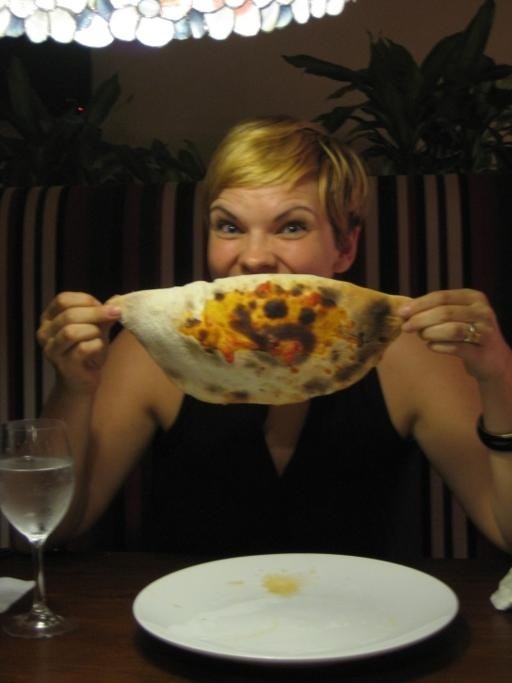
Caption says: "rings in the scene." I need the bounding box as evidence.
[461,320,480,344]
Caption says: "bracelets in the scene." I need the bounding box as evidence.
[474,412,512,455]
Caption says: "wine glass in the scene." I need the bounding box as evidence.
[0,416,76,639]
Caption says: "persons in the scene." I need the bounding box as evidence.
[6,110,512,560]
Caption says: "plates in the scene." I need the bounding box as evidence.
[132,552,460,665]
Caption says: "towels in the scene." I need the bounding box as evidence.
[0,576,36,614]
[490,568,512,611]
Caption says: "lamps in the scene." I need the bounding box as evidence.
[0,0,345,48]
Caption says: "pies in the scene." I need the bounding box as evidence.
[106,273,415,405]
